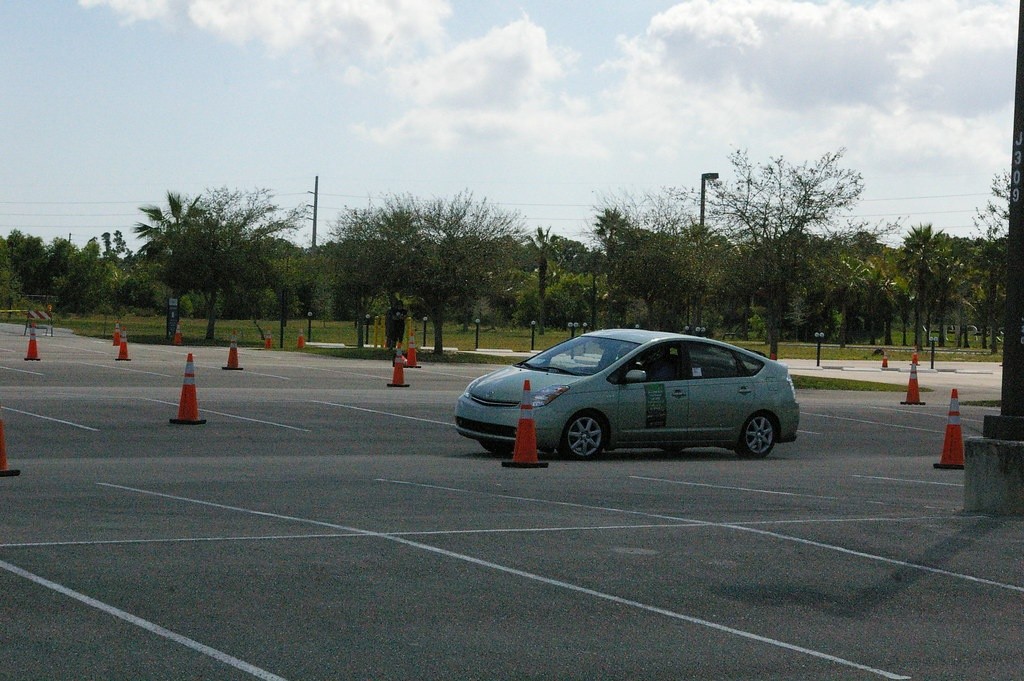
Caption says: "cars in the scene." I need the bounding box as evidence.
[453,328,803,461]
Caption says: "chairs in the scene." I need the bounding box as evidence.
[617,347,636,370]
[645,354,684,383]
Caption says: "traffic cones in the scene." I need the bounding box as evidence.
[386,343,410,388]
[404,331,422,369]
[501,379,549,468]
[221,330,243,371]
[297,328,305,348]
[0,418,20,476]
[881,347,890,370]
[933,389,965,469]
[264,327,272,350]
[170,353,207,424]
[23,323,41,361]
[112,321,120,347]
[115,327,132,361]
[172,321,185,346]
[899,353,926,405]
[392,352,421,369]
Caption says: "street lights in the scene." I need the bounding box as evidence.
[699,172,719,226]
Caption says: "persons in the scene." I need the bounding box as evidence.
[642,346,673,380]
[385,300,408,349]
[616,344,637,369]
